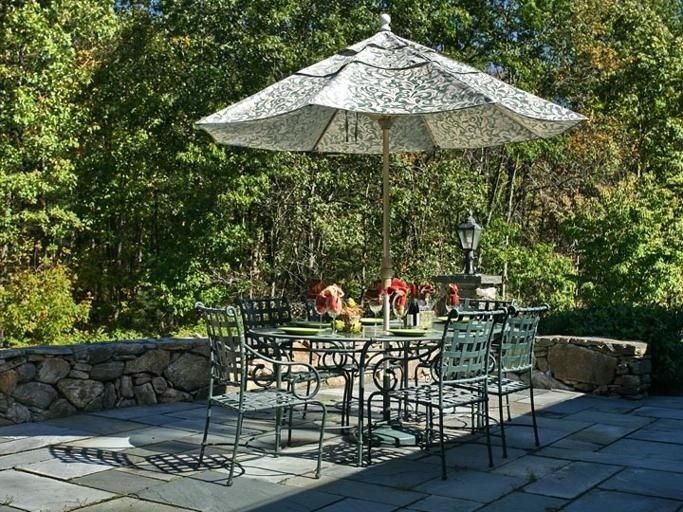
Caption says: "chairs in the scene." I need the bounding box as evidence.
[236,295,349,446]
[301,292,404,429]
[366,306,494,479]
[422,302,550,457]
[193,302,327,488]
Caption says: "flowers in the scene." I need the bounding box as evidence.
[311,278,364,333]
[366,273,460,305]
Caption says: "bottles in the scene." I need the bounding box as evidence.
[407,284,420,327]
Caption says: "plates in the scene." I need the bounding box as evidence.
[292,321,333,328]
[359,317,383,324]
[384,328,428,337]
[438,317,470,323]
[276,327,325,336]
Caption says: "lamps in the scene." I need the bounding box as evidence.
[454,208,485,275]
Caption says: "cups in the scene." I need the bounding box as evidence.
[445,295,459,313]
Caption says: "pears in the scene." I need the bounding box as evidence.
[332,320,345,331]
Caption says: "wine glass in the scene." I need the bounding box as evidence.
[367,293,383,330]
[392,293,409,329]
[327,295,343,338]
[314,294,328,329]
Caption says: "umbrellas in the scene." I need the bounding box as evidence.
[193,13,589,446]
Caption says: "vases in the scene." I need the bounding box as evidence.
[403,309,437,331]
[334,324,363,338]
